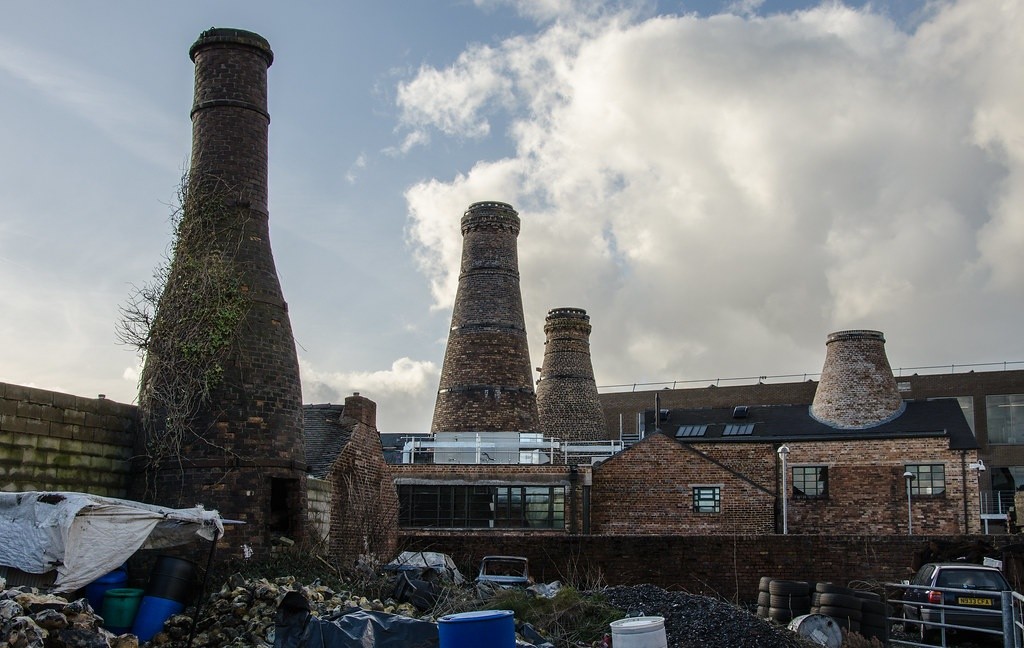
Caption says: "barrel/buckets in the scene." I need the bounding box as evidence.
[788,612,842,648]
[437,609,516,648]
[610,616,667,648]
[78,556,200,643]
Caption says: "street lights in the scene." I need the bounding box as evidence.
[903,471,915,535]
[776,444,791,534]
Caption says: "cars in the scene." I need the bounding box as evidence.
[901,562,1012,643]
[384,552,461,587]
[474,555,529,591]
